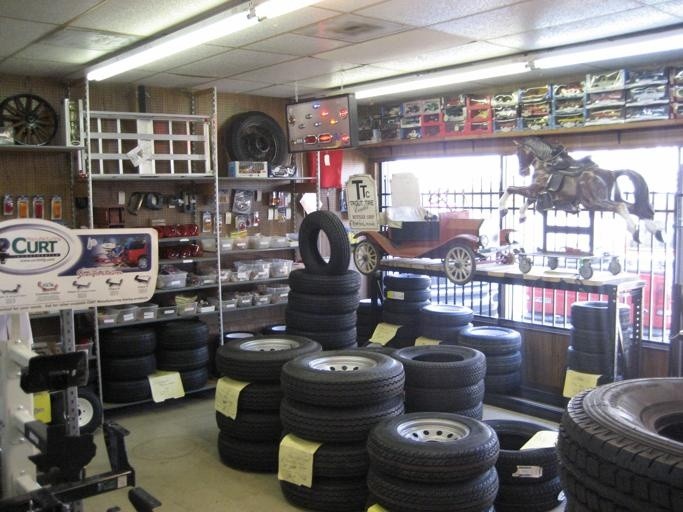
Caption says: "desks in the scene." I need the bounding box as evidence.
[374,253,646,424]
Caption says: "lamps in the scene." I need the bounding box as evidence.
[313,24,683,104]
[59,1,317,79]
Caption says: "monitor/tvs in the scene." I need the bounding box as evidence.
[285,92,360,155]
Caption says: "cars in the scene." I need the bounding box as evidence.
[114,241,150,270]
[353,169,524,286]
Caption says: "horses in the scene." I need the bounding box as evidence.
[495,135,666,247]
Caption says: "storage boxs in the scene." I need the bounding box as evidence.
[226,158,269,178]
[76,230,305,334]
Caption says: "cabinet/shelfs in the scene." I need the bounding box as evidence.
[76,80,325,411]
